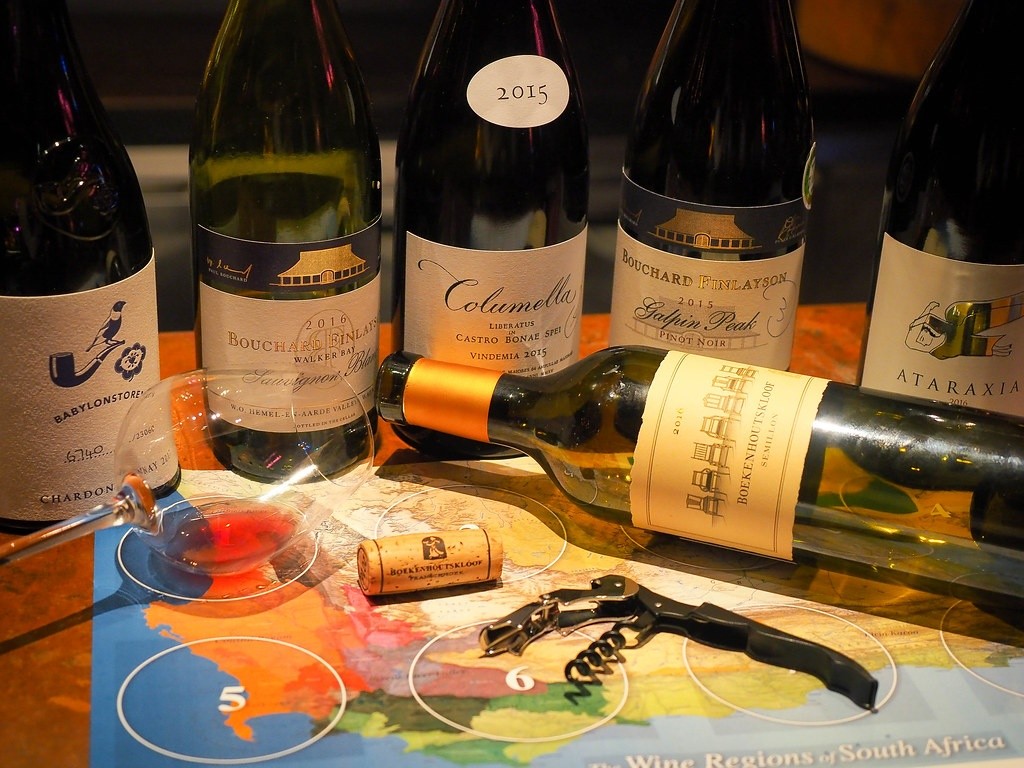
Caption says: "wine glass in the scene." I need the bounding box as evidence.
[0,364,374,577]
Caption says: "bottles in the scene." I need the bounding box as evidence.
[0,0,182,535]
[606,0,817,449]
[392,0,592,461]
[847,0,1024,489]
[375,345,1024,599]
[189,0,382,476]
